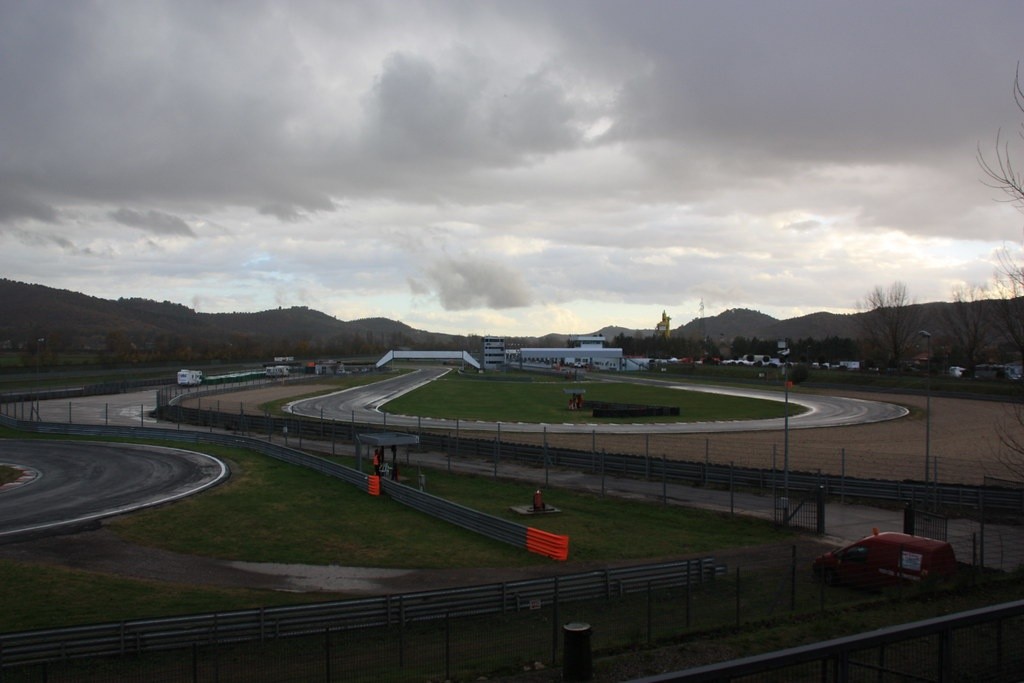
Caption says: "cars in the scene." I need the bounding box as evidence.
[574,362,583,368]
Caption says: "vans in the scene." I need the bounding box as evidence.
[812,527,959,595]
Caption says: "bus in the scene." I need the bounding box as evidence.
[266,366,289,378]
[177,369,202,387]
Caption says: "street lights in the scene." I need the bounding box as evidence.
[918,331,932,512]
[778,342,790,529]
[37,337,45,421]
[807,346,811,363]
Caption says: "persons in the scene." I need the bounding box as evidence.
[567,393,583,409]
[373,448,381,476]
